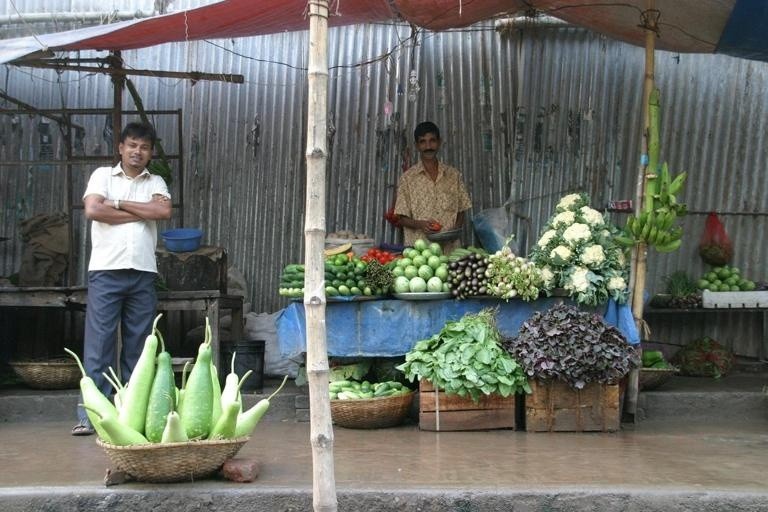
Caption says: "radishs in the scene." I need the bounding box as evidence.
[485,246,535,298]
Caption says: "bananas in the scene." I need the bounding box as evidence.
[614,162,688,252]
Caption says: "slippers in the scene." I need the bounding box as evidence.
[72,418,94,435]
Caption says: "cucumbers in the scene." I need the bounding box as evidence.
[330,380,411,400]
[279,263,383,296]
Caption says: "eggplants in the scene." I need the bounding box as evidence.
[447,252,486,300]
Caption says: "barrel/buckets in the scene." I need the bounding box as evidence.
[219,339,266,394]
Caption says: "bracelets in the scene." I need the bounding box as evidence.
[113,200,120,210]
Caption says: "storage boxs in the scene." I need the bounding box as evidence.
[696,288,768,309]
[525,378,620,435]
[419,378,516,431]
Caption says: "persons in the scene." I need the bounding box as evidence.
[69,121,175,437]
[386,120,475,251]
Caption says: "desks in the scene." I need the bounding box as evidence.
[643,307,768,371]
[288,296,631,369]
[0,287,244,392]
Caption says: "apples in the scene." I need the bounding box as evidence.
[326,248,396,269]
[698,266,755,291]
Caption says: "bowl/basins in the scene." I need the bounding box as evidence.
[159,227,206,253]
[426,229,466,241]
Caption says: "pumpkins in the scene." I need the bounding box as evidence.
[64,313,288,446]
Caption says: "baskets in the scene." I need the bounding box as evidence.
[7,358,83,388]
[330,390,417,429]
[96,436,249,483]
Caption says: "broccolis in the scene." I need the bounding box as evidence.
[535,193,628,294]
